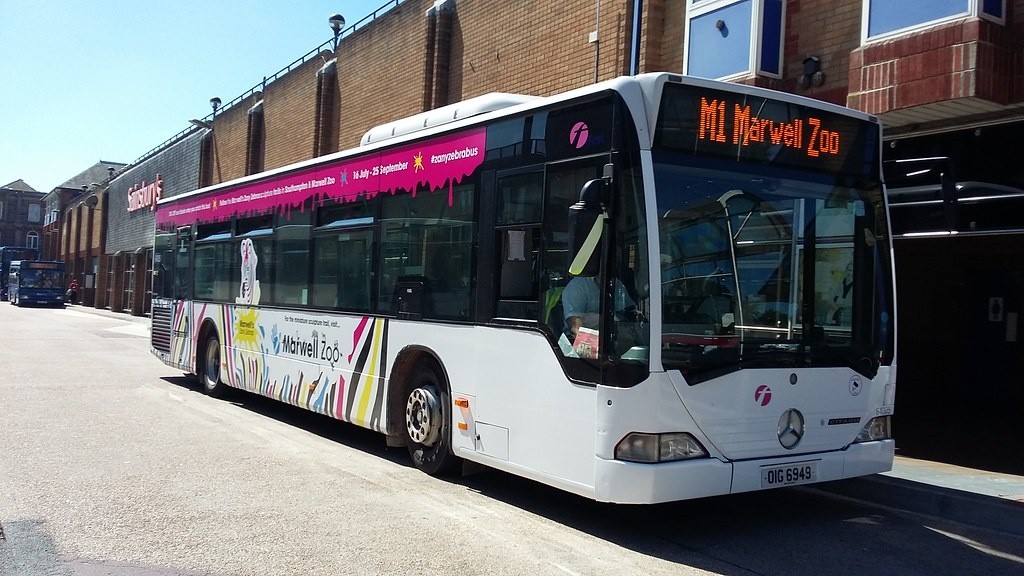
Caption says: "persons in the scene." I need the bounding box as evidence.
[672,176,739,292]
[69,279,80,305]
[42,276,53,288]
[555,243,640,346]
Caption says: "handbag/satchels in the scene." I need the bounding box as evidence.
[65,288,72,296]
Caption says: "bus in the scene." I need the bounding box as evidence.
[148,72,901,505]
[6,260,66,307]
[0,246,40,301]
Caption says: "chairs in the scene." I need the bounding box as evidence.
[541,287,566,332]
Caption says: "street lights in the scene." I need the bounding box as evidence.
[107,167,115,180]
[209,96,222,120]
[81,185,89,193]
[329,13,346,51]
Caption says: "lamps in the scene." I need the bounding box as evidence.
[210,97,221,120]
[329,14,345,51]
[81,185,88,192]
[107,167,114,178]
